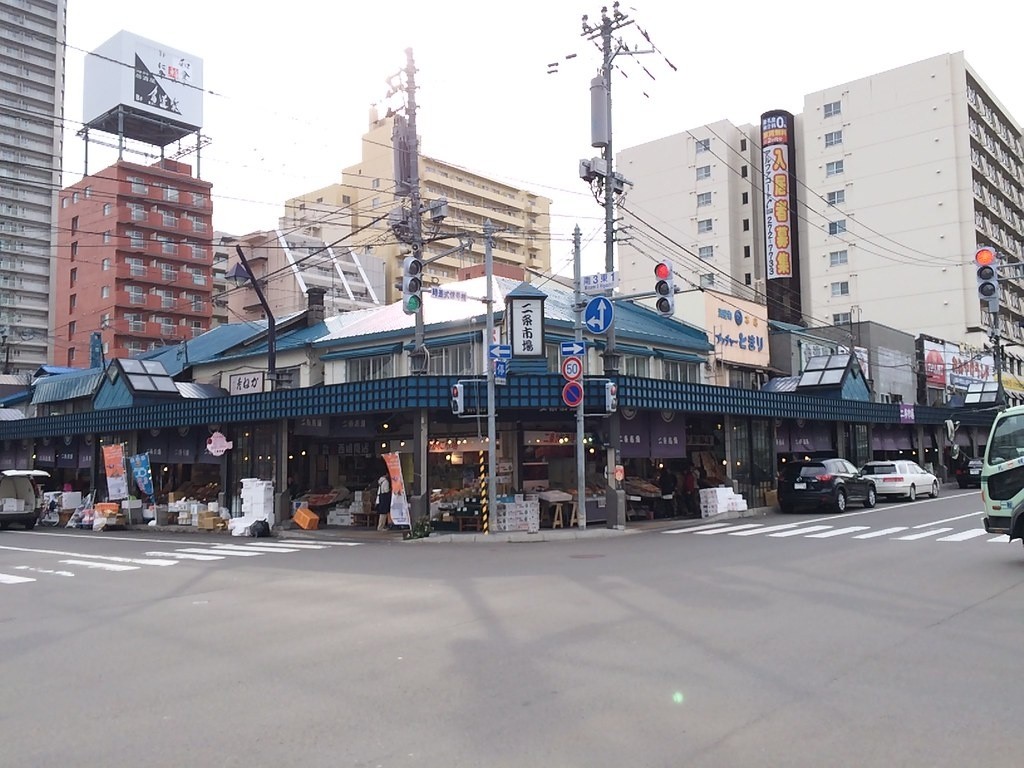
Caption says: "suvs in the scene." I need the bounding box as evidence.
[776,457,878,513]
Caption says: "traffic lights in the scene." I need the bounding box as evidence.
[450,383,464,415]
[654,260,675,318]
[403,254,427,315]
[976,244,1003,302]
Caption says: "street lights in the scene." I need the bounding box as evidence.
[225,242,277,391]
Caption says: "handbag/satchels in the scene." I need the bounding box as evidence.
[697,478,703,489]
[250,518,270,538]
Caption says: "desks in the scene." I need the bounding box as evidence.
[353,510,378,529]
[456,516,483,531]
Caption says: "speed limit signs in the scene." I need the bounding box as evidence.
[562,356,583,382]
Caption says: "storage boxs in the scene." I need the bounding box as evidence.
[699,487,748,518]
[291,501,319,530]
[44,491,82,526]
[327,488,379,526]
[765,489,778,507]
[83,499,142,529]
[0,497,25,513]
[228,478,274,536]
[142,491,232,530]
[497,480,549,533]
[431,488,484,522]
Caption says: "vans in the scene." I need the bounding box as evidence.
[982,405,1024,547]
[0,468,51,530]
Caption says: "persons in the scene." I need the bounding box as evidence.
[377,471,391,531]
[659,463,701,518]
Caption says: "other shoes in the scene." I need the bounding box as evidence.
[377,527,388,531]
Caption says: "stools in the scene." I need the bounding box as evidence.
[541,503,563,529]
[566,501,579,527]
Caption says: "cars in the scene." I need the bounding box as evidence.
[859,459,940,501]
[956,456,1002,489]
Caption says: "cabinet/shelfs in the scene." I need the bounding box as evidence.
[542,480,663,526]
